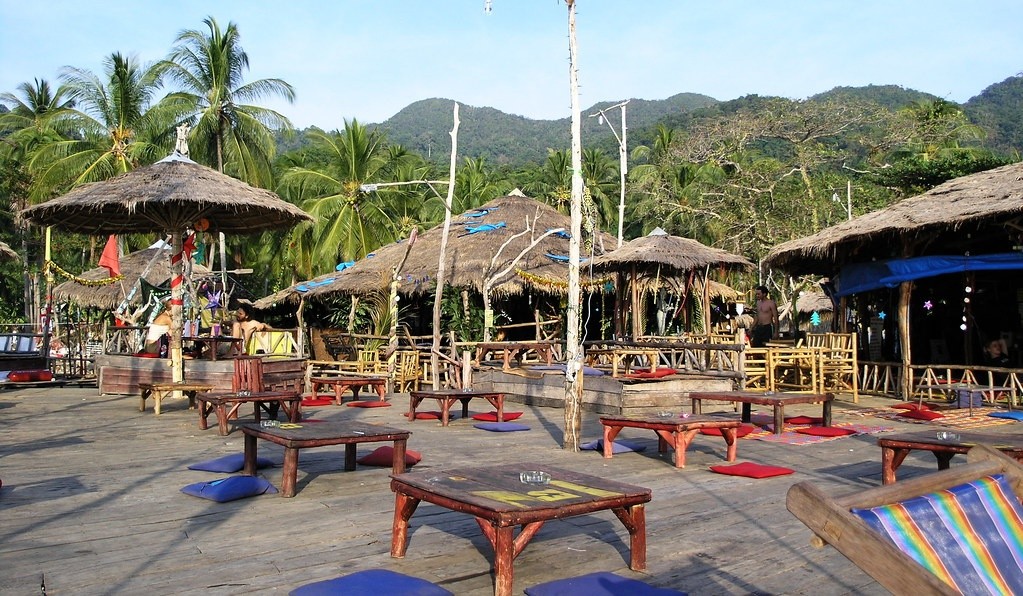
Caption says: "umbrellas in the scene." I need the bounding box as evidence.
[585,226,754,340]
[14,152,310,396]
[50,239,218,311]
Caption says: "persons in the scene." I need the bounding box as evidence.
[985,340,1021,389]
[146,299,181,353]
[207,303,274,358]
[745,286,779,386]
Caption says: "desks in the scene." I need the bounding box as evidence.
[597,414,738,467]
[309,376,387,405]
[477,343,552,370]
[163,334,244,360]
[196,392,301,436]
[241,421,411,497]
[137,383,213,417]
[586,348,659,377]
[690,387,838,433]
[409,390,508,426]
[388,461,652,596]
[917,382,1015,413]
[877,428,1022,487]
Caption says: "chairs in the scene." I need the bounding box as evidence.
[359,350,381,375]
[395,351,420,393]
[737,330,857,403]
[785,441,1023,596]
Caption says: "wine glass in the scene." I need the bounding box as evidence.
[213,325,220,338]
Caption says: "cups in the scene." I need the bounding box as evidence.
[191,323,197,338]
